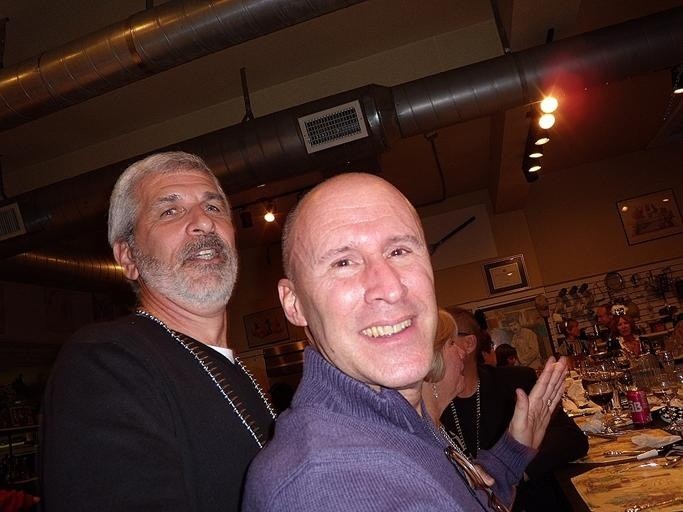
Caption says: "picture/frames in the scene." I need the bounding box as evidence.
[615,188,682,248]
[474,296,555,372]
[478,252,530,299]
[242,306,290,350]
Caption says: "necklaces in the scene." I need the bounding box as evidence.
[135,292,278,449]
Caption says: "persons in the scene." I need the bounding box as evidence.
[503,317,541,370]
[240,172,568,511]
[420,305,589,512]
[610,314,651,356]
[36,151,277,510]
[558,317,591,360]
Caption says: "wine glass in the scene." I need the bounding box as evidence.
[652,349,683,432]
[580,364,616,437]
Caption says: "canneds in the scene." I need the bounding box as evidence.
[627,389,653,425]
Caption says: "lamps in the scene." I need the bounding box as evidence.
[520,118,550,183]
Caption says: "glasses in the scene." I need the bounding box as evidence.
[443,446,509,512]
